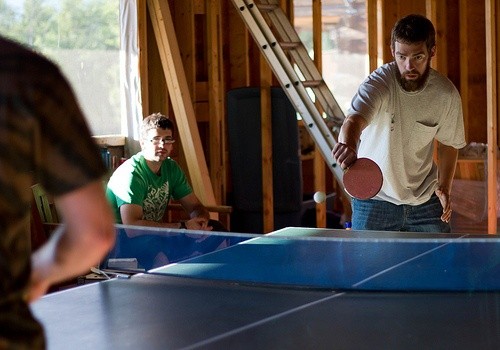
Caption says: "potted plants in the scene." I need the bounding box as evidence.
[457,142,487,181]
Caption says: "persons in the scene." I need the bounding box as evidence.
[0,28,117,350]
[104,113,227,270]
[332,14,466,235]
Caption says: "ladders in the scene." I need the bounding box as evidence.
[231,0,361,197]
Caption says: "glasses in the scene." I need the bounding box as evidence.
[144,136,176,143]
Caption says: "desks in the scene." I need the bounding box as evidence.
[29,226,500,350]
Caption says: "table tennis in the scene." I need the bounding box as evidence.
[314,192,326,203]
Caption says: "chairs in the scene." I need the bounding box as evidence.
[164,204,232,233]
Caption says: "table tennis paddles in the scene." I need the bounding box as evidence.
[343,158,383,201]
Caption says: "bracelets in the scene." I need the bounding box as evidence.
[178,220,188,230]
